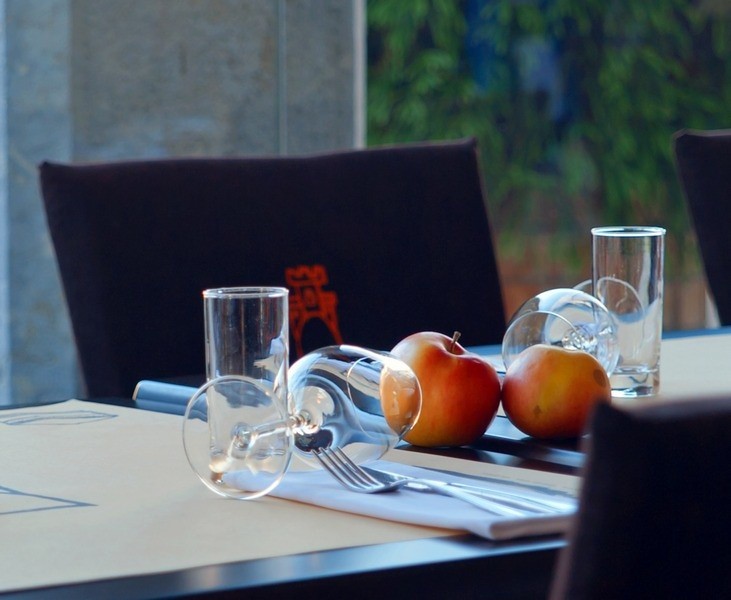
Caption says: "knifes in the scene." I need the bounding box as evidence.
[346,462,576,513]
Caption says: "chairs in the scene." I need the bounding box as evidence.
[38,139,508,407]
[554,395,731,600]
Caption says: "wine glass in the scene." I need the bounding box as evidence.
[502,277,646,379]
[182,344,422,501]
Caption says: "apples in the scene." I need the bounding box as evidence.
[501,343,610,438]
[380,330,501,447]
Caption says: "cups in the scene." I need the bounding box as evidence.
[202,286,289,486]
[591,226,666,398]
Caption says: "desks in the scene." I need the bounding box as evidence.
[0,327,731,600]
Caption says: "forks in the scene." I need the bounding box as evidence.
[312,447,526,517]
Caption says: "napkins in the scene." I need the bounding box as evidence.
[221,463,578,539]
